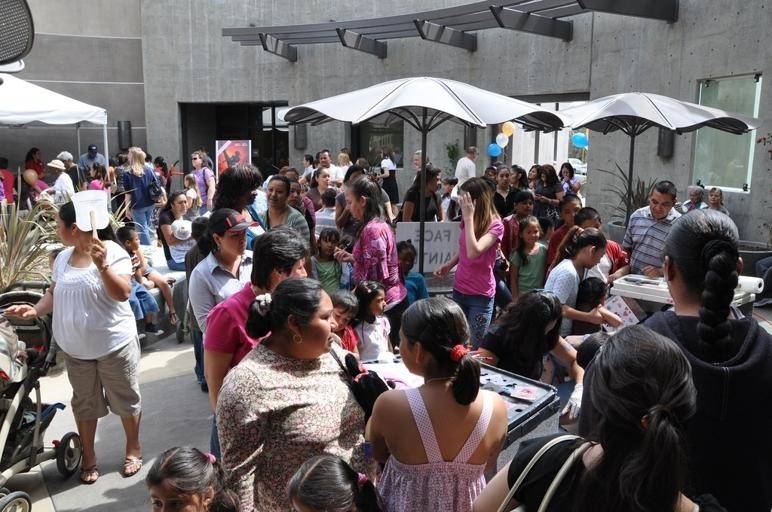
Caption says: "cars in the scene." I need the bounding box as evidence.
[568,157,586,176]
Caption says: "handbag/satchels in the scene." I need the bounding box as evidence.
[147,167,163,202]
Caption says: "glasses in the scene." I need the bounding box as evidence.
[190,156,200,161]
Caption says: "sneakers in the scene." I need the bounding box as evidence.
[146,324,166,336]
[136,327,147,341]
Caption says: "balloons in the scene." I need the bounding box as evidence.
[571,133,589,148]
[486,121,515,158]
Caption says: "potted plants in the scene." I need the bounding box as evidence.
[592,158,662,251]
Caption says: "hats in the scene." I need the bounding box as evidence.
[46,158,67,173]
[207,207,260,238]
[87,144,99,153]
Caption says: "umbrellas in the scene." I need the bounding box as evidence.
[557,91,765,224]
[283,74,562,269]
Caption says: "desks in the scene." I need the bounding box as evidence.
[606,267,757,320]
[347,343,563,477]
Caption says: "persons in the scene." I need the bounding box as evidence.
[1,141,771,511]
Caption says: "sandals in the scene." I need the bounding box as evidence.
[78,462,99,485]
[169,311,181,327]
[123,454,143,478]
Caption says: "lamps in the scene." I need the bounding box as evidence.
[656,122,675,163]
[463,124,478,152]
[293,122,308,150]
[117,120,133,152]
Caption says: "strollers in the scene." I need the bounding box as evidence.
[175,298,193,343]
[0,278,82,512]
